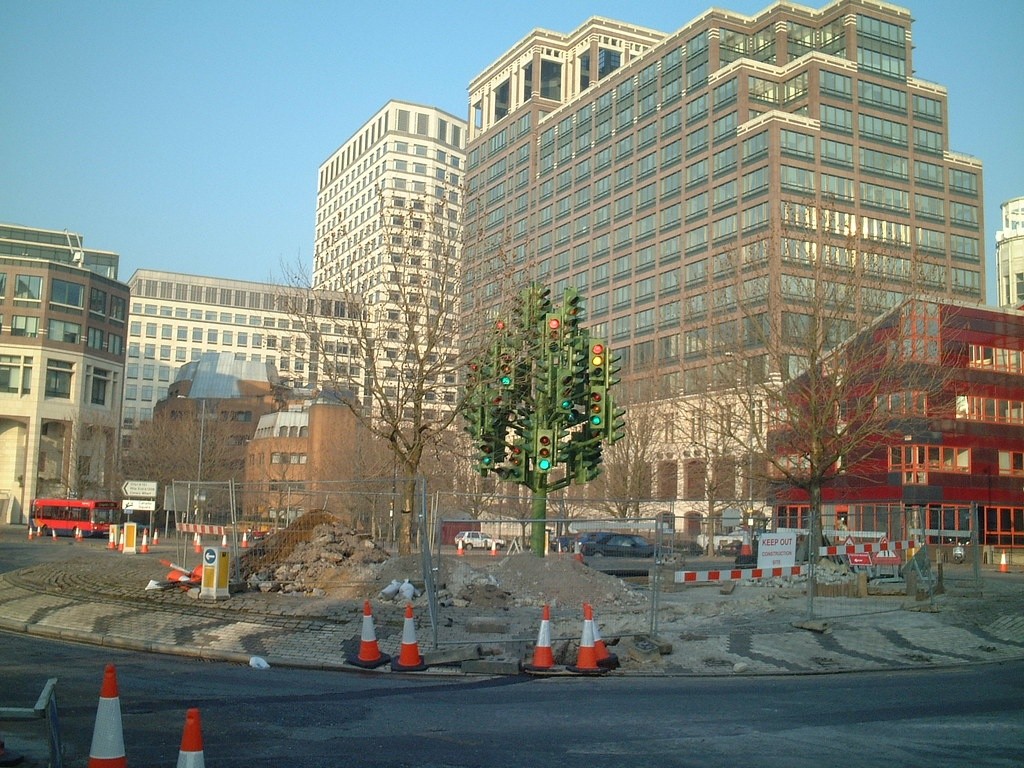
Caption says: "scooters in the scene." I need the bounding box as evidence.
[949,538,971,564]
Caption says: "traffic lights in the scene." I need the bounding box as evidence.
[607,400,625,446]
[462,279,586,482]
[574,445,596,484]
[606,347,622,390]
[589,386,606,430]
[589,338,605,382]
[591,441,603,467]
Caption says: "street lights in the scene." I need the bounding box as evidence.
[177,396,206,524]
[725,351,754,536]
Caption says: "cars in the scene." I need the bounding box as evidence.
[662,539,703,556]
[550,531,680,558]
[719,542,743,556]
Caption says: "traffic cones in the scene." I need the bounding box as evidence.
[117,529,124,550]
[51,528,58,541]
[154,528,159,545]
[37,526,42,537]
[525,605,564,672]
[575,537,585,566]
[391,603,426,670]
[77,530,84,543]
[192,532,198,546]
[457,540,465,557]
[1000,549,1008,573]
[565,605,605,673]
[137,528,148,553]
[491,540,497,556]
[194,534,202,554]
[240,530,249,548]
[106,528,116,549]
[28,525,34,540]
[176,707,206,768]
[582,603,609,662]
[75,525,79,539]
[86,661,130,768]
[222,535,228,549]
[167,564,203,591]
[345,601,392,668]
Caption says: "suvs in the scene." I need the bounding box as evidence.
[455,530,505,551]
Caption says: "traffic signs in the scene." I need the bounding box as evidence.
[122,480,158,499]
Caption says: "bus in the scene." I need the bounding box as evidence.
[28,498,121,538]
